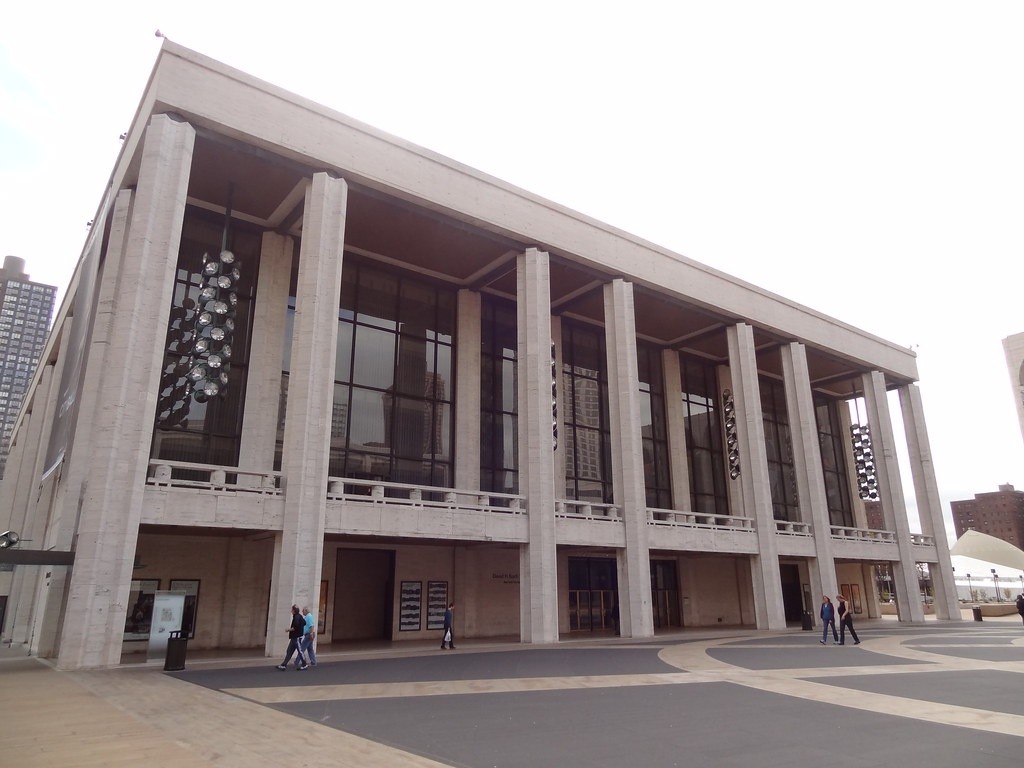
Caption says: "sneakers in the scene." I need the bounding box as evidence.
[275,665,287,671]
[297,665,309,670]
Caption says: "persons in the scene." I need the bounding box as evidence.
[611,600,620,636]
[1016,595,1024,625]
[292,606,317,667]
[836,594,860,646]
[275,604,309,672]
[441,603,456,649]
[820,596,838,645]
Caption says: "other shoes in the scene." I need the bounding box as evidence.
[854,641,861,645]
[820,640,827,645]
[308,664,318,667]
[833,642,838,644]
[450,646,456,649]
[440,646,448,649]
[290,662,298,666]
[838,642,844,645]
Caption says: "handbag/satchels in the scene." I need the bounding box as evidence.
[444,629,451,642]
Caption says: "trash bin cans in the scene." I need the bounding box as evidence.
[164,631,188,671]
